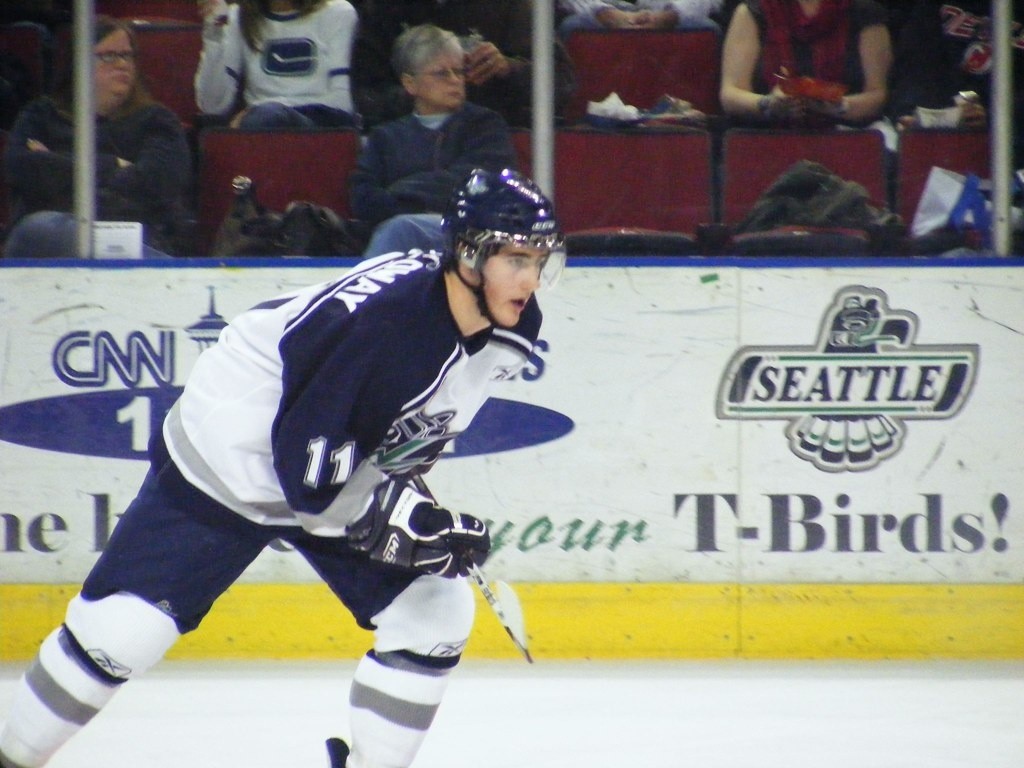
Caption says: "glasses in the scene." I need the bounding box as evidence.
[93,50,136,63]
[414,69,465,81]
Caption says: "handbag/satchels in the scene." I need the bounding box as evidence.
[911,165,1024,252]
[242,200,352,258]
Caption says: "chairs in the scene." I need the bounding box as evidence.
[0,0,1024,259]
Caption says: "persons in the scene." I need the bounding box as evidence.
[0,0,1024,258]
[0,161,561,768]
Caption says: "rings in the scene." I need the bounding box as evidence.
[486,59,494,66]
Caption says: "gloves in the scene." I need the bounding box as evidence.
[345,475,490,578]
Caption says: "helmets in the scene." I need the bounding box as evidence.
[440,167,566,271]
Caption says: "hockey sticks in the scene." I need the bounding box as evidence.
[412,469,537,667]
